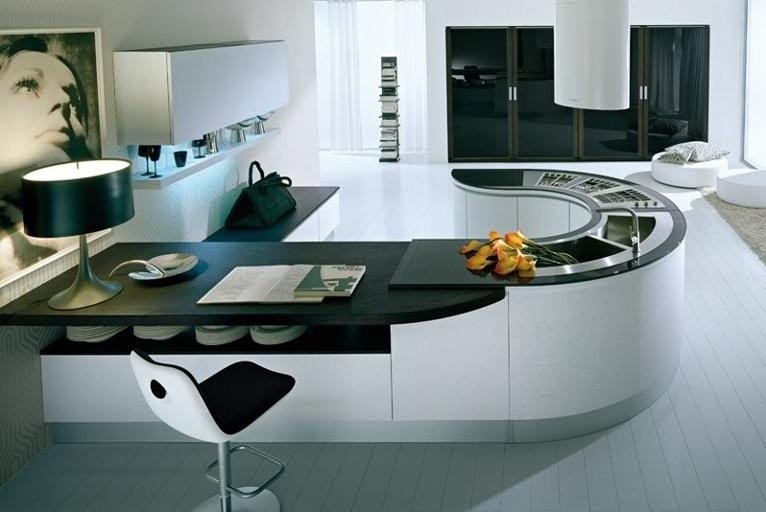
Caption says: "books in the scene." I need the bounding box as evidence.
[195,265,325,304]
[294,265,367,297]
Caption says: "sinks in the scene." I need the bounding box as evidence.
[518,235,624,266]
[593,214,657,246]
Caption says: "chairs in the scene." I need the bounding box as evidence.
[130,349,297,512]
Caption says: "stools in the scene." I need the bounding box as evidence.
[652,152,766,209]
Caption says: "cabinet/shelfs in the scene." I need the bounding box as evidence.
[113,39,287,191]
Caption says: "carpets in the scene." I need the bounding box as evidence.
[696,159,766,266]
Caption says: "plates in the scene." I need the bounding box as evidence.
[65,325,309,346]
[108,254,199,281]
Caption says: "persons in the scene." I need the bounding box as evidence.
[1,36,89,201]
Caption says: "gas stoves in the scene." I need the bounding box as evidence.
[537,170,665,209]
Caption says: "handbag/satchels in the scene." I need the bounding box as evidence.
[226,161,297,230]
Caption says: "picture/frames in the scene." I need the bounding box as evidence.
[1,26,113,312]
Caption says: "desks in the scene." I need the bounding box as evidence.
[204,186,340,242]
[1,237,504,460]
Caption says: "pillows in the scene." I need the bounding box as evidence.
[656,140,733,162]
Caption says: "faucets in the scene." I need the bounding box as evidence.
[594,205,640,261]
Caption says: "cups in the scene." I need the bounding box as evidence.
[174,151,187,167]
[192,140,206,159]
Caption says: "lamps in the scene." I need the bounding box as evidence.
[20,155,135,310]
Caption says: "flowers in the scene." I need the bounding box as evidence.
[461,227,577,278]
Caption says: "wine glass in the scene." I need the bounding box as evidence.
[138,145,162,179]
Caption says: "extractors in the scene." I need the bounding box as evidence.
[553,0,630,110]
